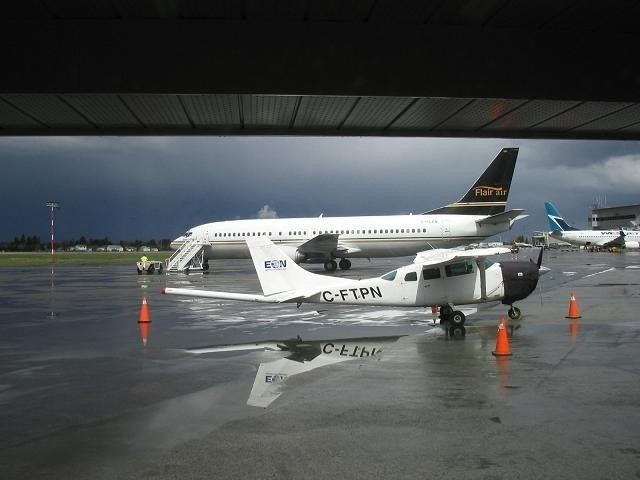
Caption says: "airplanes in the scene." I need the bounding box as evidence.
[184,327,466,409]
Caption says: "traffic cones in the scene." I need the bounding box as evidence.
[138,323,152,348]
[492,316,513,356]
[137,294,152,323]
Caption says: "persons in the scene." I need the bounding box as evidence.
[512,242,519,258]
[140,253,148,270]
